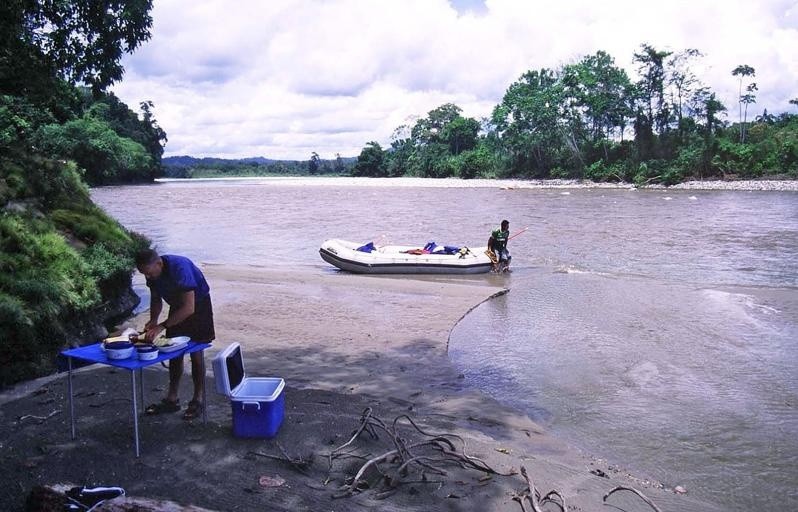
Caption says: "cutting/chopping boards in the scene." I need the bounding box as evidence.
[105,334,153,346]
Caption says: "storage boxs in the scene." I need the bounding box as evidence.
[212,341,287,439]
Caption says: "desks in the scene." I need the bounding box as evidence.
[60,342,212,458]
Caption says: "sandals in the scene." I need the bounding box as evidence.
[145,398,181,415]
[183,400,203,420]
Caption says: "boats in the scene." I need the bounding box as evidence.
[319,238,492,274]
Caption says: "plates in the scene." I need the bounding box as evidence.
[156,334,192,353]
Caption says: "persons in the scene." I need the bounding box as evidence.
[133,249,215,420]
[485,220,512,272]
